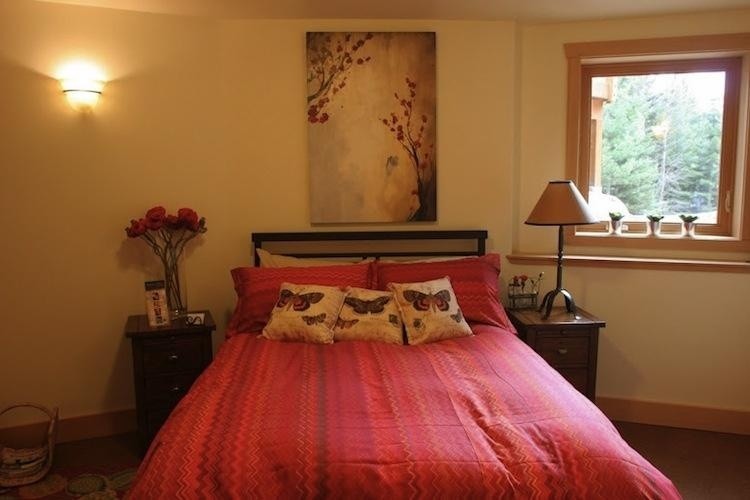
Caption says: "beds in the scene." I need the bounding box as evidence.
[128,230,683,499]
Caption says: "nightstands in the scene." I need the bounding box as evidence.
[507,305,605,405]
[126,308,216,451]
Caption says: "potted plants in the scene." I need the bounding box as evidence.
[607,211,625,235]
[680,213,698,237]
[646,215,664,236]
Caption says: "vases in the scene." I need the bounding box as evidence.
[160,249,188,321]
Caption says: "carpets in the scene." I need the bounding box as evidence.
[0,461,140,500]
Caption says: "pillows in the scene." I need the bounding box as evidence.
[333,288,404,347]
[255,247,367,268]
[226,261,374,346]
[387,276,473,345]
[375,253,506,331]
[380,254,475,264]
[258,282,346,346]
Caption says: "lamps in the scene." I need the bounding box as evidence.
[524,180,601,321]
[58,78,105,114]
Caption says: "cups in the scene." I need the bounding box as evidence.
[681,223,695,237]
[646,221,660,236]
[609,221,622,235]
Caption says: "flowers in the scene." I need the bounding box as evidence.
[126,206,207,310]
